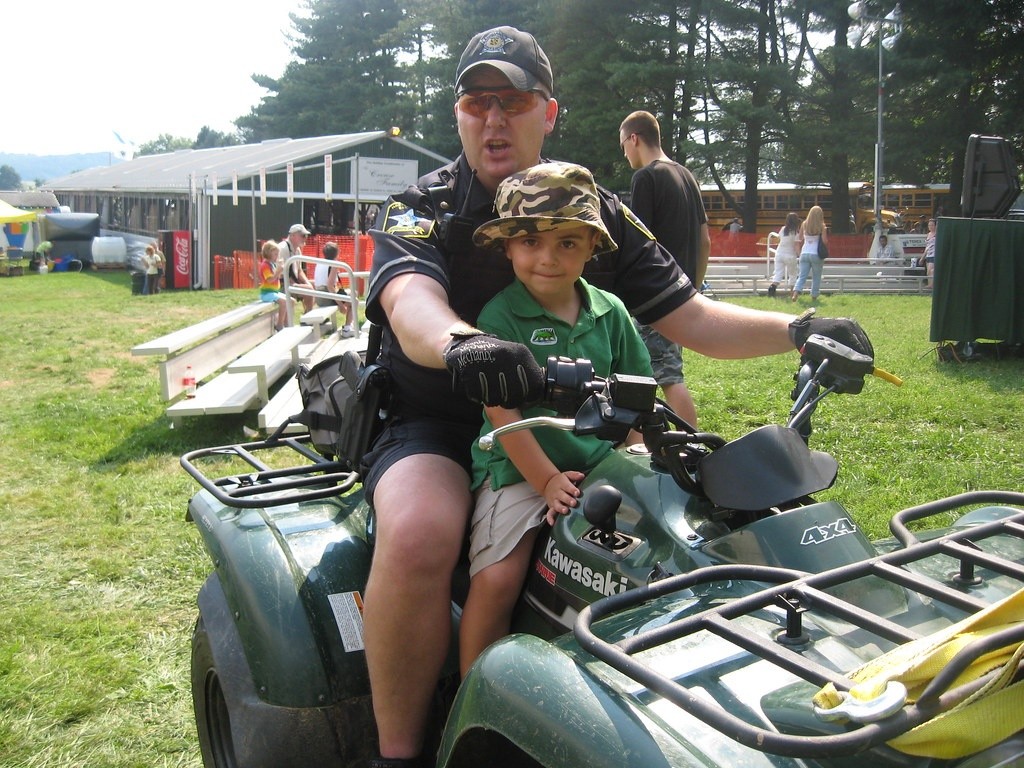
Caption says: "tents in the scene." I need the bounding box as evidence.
[0,199,46,265]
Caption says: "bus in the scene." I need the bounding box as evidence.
[699,182,952,237]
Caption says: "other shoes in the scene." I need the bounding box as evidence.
[769,285,776,297]
[274,323,285,332]
[342,326,355,338]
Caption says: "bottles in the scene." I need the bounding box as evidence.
[185,366,196,398]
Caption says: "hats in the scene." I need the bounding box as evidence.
[452,26,553,94]
[289,224,312,235]
[472,162,620,259]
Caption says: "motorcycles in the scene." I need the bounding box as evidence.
[180,332,1023,768]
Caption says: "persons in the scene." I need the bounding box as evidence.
[728,217,745,256]
[918,219,936,291]
[620,111,713,437]
[142,243,166,295]
[459,162,658,688]
[848,208,858,233]
[768,206,827,300]
[314,241,359,339]
[259,240,295,332]
[278,224,316,314]
[871,236,897,266]
[357,26,875,768]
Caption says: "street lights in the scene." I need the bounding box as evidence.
[847,3,904,235]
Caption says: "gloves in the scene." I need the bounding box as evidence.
[787,308,874,396]
[444,332,547,410]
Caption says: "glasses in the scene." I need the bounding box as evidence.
[296,232,308,239]
[458,92,551,120]
[619,132,644,150]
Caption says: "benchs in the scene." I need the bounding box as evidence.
[131,293,382,434]
[699,255,933,296]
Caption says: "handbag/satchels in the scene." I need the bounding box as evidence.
[333,289,347,305]
[296,348,387,458]
[158,267,164,275]
[818,225,828,261]
